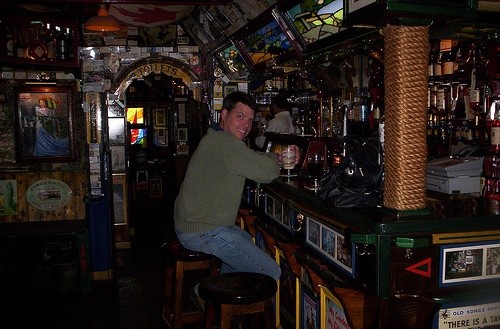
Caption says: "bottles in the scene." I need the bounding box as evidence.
[1,20,76,61]
[429,24,500,218]
[244,52,384,192]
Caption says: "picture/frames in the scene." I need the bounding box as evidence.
[13,86,74,163]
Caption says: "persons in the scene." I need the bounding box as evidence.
[252,95,294,153]
[172,90,282,316]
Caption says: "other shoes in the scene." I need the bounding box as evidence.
[189,283,206,316]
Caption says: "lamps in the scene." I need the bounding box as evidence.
[86,4,120,31]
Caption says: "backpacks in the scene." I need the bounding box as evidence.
[318,135,382,208]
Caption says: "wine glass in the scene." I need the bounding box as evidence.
[281,150,296,183]
[306,152,327,189]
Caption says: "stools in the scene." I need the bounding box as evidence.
[198,272,278,329]
[161,240,216,329]
